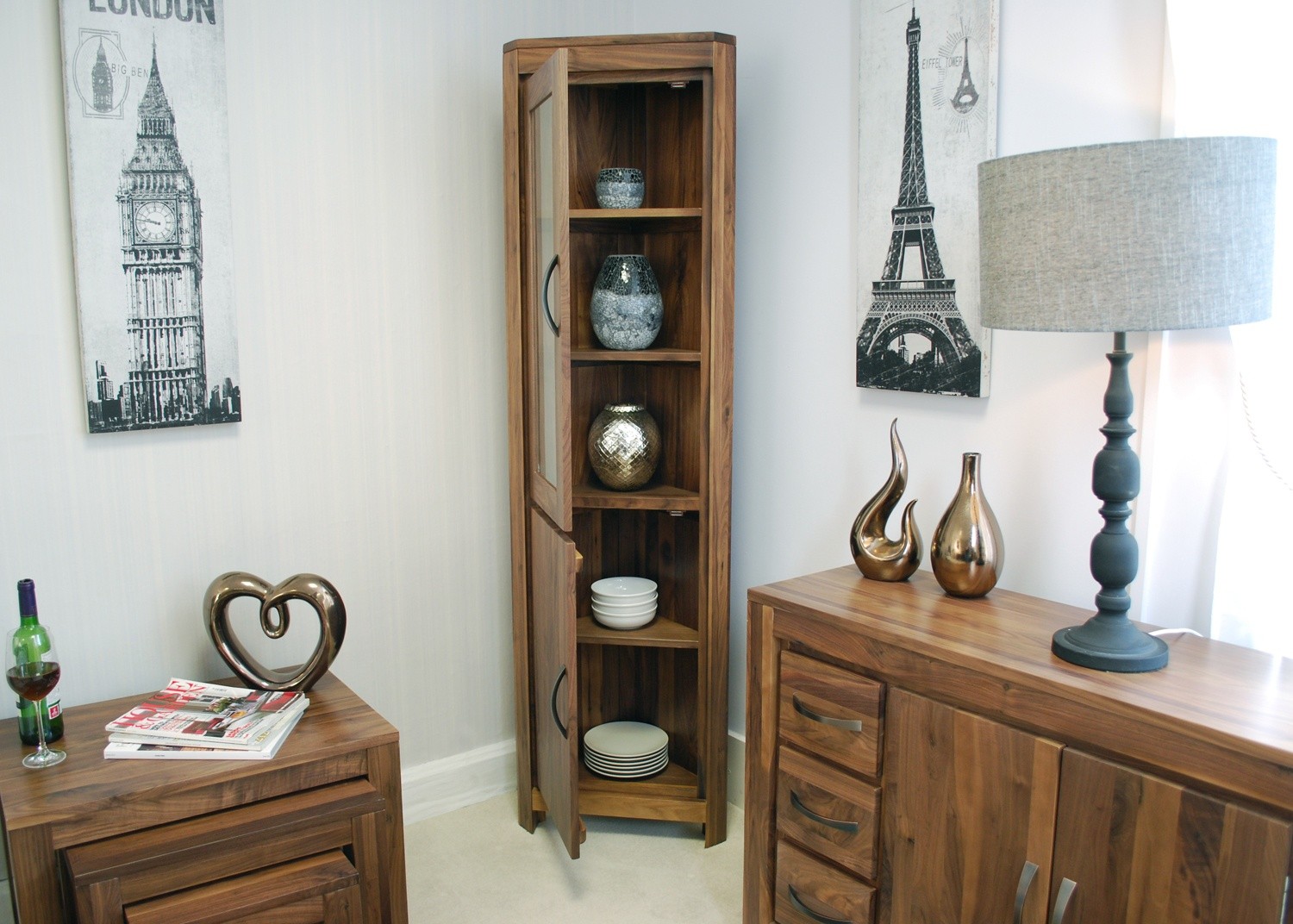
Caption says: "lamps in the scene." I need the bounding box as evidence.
[977,135,1276,672]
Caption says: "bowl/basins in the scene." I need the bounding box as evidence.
[590,576,659,631]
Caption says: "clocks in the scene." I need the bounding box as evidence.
[133,200,177,244]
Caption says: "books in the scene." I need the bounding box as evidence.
[105,678,305,745]
[109,698,310,751]
[104,710,304,760]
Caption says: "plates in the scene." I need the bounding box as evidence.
[582,720,671,781]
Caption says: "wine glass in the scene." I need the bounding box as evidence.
[5,625,68,769]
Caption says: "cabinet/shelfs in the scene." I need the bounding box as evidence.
[0,664,408,924]
[743,563,1293,924]
[502,32,737,861]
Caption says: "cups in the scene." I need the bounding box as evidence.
[596,168,645,209]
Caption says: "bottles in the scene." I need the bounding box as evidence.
[932,452,1005,599]
[588,254,664,351]
[586,402,662,492]
[12,578,65,745]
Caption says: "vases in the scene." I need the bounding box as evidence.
[596,168,643,208]
[587,402,663,490]
[589,256,663,350]
